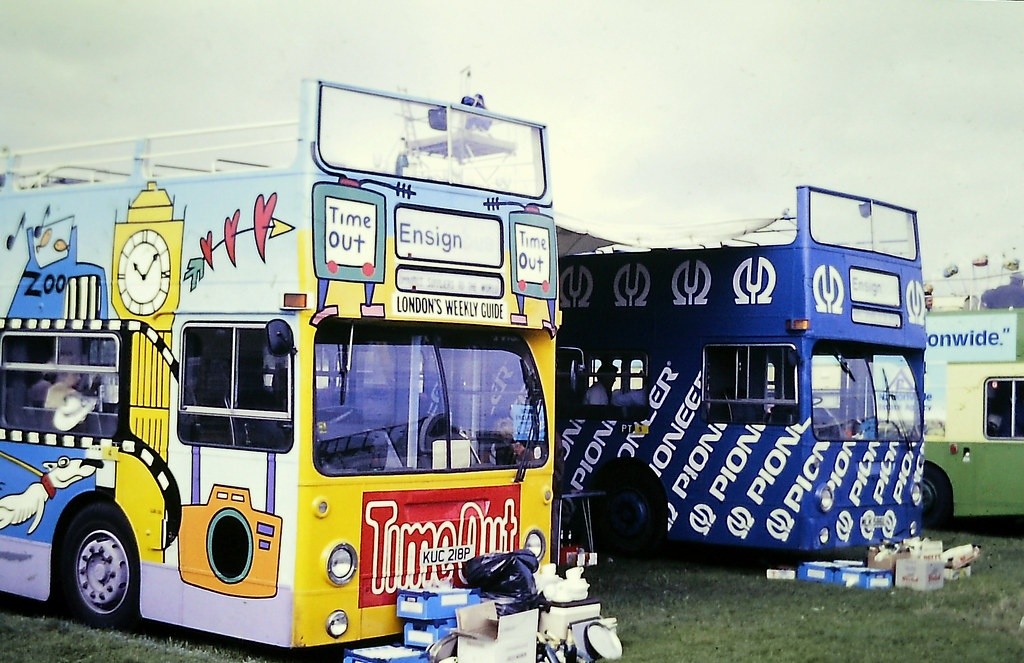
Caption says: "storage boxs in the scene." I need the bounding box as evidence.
[765,539,979,592]
[344,578,617,663]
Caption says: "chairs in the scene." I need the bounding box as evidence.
[185,355,231,434]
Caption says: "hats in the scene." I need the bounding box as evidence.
[53,393,97,431]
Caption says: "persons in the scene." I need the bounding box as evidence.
[30,357,88,411]
[583,365,644,405]
[489,419,525,465]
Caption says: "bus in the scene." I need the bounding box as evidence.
[363,185,925,558]
[1,79,589,653]
[764,307,1023,526]
